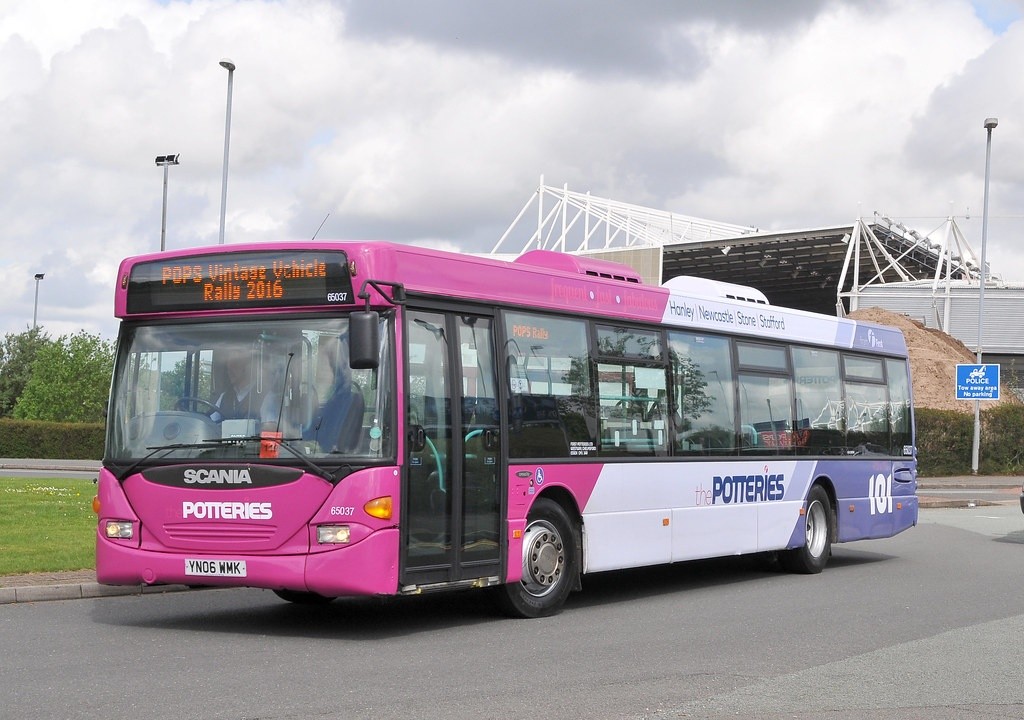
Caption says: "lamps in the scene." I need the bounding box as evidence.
[873,248,880,257]
[885,252,892,261]
[758,259,768,269]
[818,277,831,290]
[899,259,908,269]
[840,233,850,245]
[790,266,802,280]
[721,246,732,257]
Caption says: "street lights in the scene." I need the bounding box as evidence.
[969,117,1001,476]
[32,272,46,330]
[529,345,549,374]
[707,370,731,426]
[155,153,180,250]
[217,57,237,244]
[274,350,295,433]
[459,316,487,400]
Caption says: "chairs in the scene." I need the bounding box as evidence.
[300,382,319,439]
[310,381,365,454]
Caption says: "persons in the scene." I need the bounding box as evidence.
[204,349,281,437]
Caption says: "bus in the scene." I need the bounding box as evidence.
[90,236,921,619]
